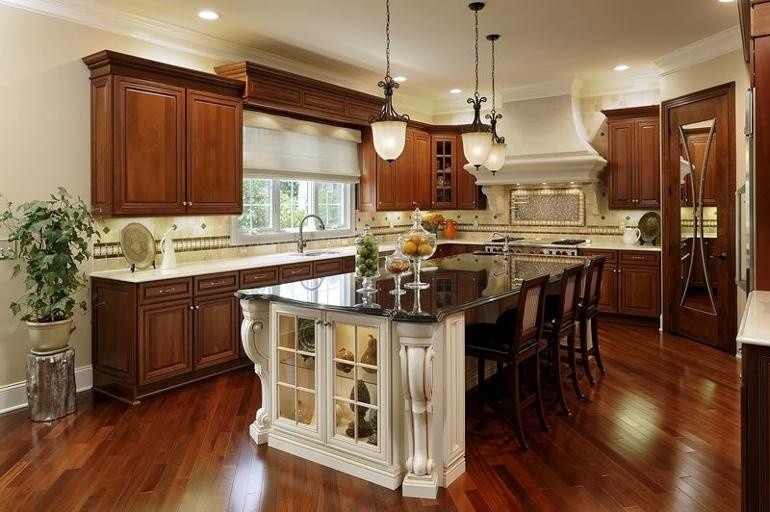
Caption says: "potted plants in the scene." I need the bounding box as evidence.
[0,188,110,356]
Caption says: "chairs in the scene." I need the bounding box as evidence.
[466,252,605,452]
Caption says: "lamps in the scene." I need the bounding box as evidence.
[368,1,410,164]
[459,2,494,174]
[484,33,506,174]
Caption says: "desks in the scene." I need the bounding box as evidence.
[736,291,770,511]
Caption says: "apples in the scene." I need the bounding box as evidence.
[402,233,434,257]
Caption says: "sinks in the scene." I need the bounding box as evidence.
[291,250,337,259]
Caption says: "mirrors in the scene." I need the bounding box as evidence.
[670,117,728,317]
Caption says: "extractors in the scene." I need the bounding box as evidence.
[463,151,606,186]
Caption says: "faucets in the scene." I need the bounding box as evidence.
[492,231,509,254]
[297,214,325,253]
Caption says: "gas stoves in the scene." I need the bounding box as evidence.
[491,237,591,246]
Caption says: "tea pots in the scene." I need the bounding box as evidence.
[623,228,641,243]
[623,228,642,246]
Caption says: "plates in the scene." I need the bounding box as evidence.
[121,222,156,269]
[638,212,661,241]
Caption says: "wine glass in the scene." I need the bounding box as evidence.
[385,254,410,314]
[355,237,381,311]
[405,260,430,289]
[399,207,437,315]
[355,272,380,293]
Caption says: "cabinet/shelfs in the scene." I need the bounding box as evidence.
[577,248,661,317]
[454,124,485,210]
[601,104,659,210]
[681,238,690,293]
[240,255,355,367]
[426,124,457,212]
[358,120,430,212]
[81,50,244,217]
[681,127,715,204]
[89,272,241,407]
[375,266,489,313]
[691,237,718,288]
[234,252,595,500]
